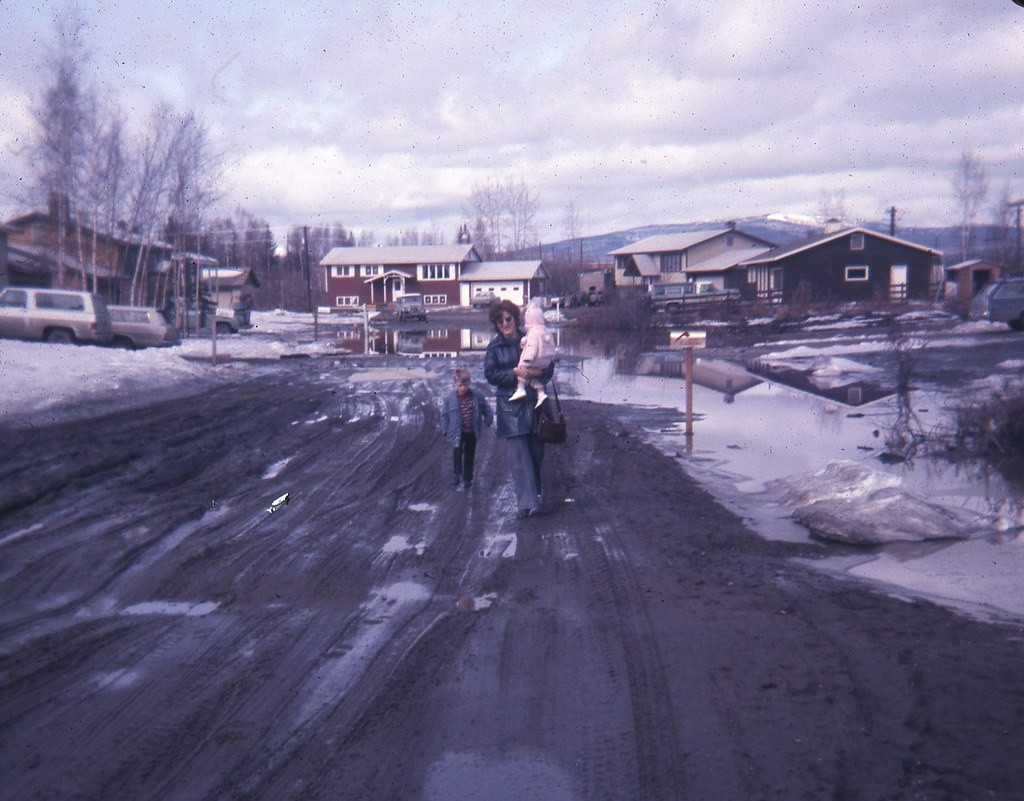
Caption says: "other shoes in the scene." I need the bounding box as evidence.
[517,509,531,519]
[464,481,472,487]
[451,477,459,485]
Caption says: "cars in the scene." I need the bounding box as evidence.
[106,304,179,351]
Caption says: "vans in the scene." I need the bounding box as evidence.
[0,286,114,345]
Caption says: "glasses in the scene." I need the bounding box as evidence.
[494,316,514,325]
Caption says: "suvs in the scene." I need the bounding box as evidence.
[396,292,427,322]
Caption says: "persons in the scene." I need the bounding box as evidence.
[441,369,494,489]
[484,299,555,520]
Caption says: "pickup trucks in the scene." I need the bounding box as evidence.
[968,278,1024,332]
[585,291,607,306]
[472,292,500,307]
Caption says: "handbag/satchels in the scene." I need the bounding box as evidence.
[537,411,567,444]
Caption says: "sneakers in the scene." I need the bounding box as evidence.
[534,394,548,410]
[508,390,526,402]
